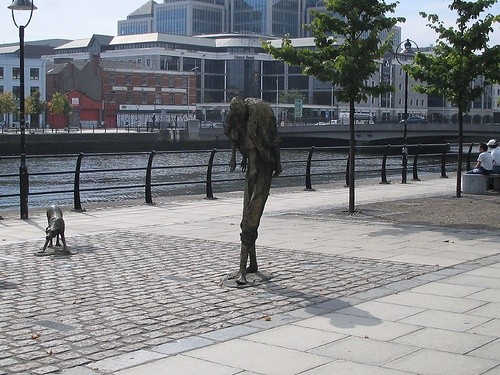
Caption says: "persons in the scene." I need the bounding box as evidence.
[467,144,493,175]
[487,139,500,175]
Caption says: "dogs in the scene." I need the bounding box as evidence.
[38,205,70,253]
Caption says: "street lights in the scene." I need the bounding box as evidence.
[8,0,38,218]
[188,67,199,116]
[396,39,419,183]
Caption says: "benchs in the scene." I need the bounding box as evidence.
[463,174,500,194]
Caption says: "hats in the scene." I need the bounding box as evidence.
[486,139,498,145]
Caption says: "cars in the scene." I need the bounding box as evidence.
[199,120,214,128]
[314,119,338,126]
[398,115,429,124]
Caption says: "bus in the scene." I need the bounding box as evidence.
[338,108,375,124]
